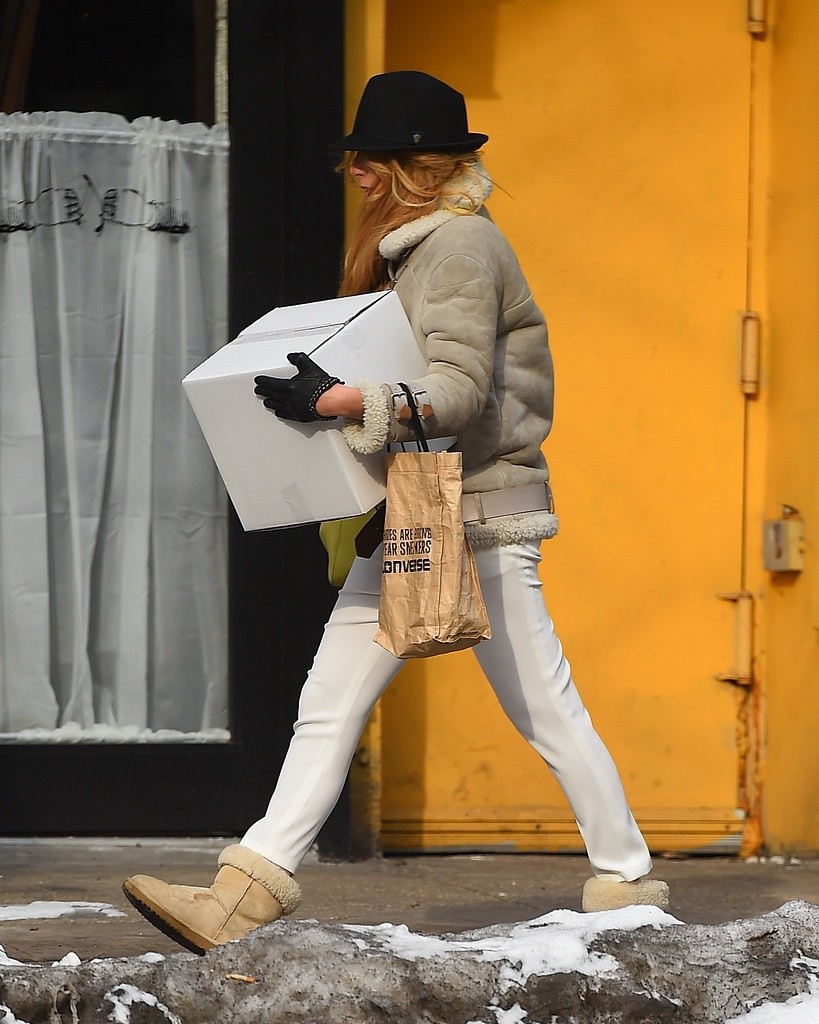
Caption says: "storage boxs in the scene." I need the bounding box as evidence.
[180,288,460,533]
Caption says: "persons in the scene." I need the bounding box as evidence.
[122,70,669,957]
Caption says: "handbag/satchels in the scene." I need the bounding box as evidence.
[374,383,494,661]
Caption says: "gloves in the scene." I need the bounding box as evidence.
[253,352,345,423]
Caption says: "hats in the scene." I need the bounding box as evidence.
[342,70,490,157]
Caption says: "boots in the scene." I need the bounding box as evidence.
[582,876,670,917]
[121,841,306,958]
